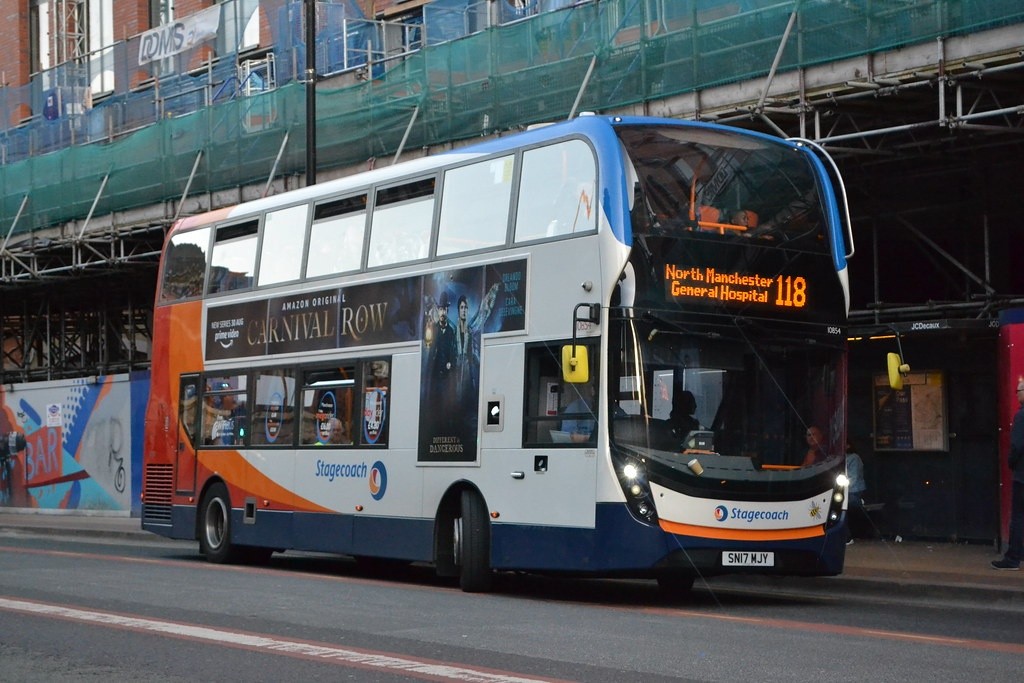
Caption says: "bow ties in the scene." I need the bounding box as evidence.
[441,325,447,329]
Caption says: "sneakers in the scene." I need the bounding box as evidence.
[846,535,854,546]
[991,559,1020,570]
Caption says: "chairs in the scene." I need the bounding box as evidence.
[688,205,726,233]
[745,208,760,229]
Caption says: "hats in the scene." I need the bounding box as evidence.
[437,291,451,307]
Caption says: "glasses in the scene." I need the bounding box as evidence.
[461,305,468,309]
[1015,390,1022,394]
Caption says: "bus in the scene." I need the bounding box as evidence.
[138,110,912,594]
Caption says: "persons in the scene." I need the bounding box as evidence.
[799,422,830,470]
[187,383,248,446]
[845,440,868,546]
[725,209,752,238]
[324,417,352,444]
[662,390,701,442]
[989,374,1024,571]
[425,291,476,402]
[561,374,629,443]
